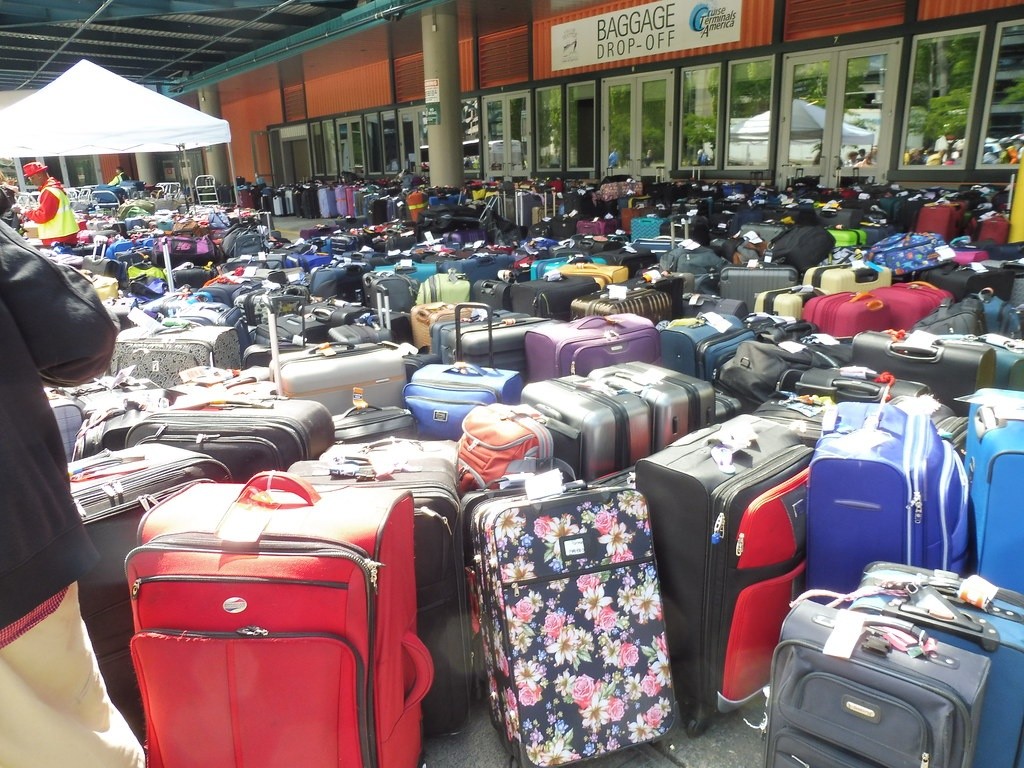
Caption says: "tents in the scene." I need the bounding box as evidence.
[729,98,874,165]
[0,60,239,206]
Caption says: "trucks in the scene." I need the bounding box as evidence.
[488,140,522,171]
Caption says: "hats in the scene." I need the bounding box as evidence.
[23,162,48,177]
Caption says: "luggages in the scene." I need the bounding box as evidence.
[31,172,1023,767]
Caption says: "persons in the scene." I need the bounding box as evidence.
[697,146,709,165]
[0,170,20,230]
[0,221,146,768]
[904,134,1024,165]
[116,167,129,181]
[844,144,877,167]
[644,150,652,167]
[610,148,619,169]
[12,162,81,249]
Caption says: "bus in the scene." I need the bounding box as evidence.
[463,139,480,168]
